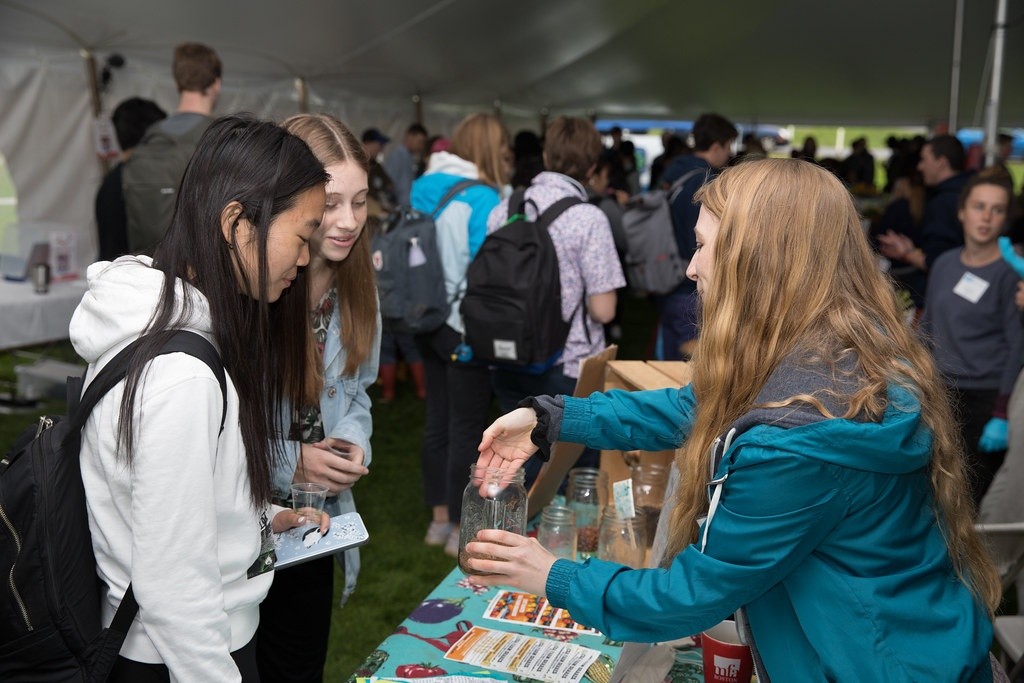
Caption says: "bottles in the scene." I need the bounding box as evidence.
[457,463,528,577]
[566,468,611,551]
[597,504,648,569]
[408,237,427,266]
[631,462,671,547]
[537,506,578,562]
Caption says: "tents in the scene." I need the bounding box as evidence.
[0,0,1024,281]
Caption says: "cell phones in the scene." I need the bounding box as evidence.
[274,512,370,570]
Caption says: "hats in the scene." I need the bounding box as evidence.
[111,97,167,152]
[363,128,390,145]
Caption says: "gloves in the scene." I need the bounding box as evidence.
[978,416,1010,452]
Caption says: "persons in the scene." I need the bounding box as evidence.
[95,42,641,557]
[63,114,383,682]
[650,114,1024,515]
[464,159,997,683]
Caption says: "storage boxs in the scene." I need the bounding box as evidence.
[601,361,698,508]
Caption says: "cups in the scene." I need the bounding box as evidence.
[701,620,754,683]
[31,262,49,292]
[326,434,362,462]
[292,482,330,530]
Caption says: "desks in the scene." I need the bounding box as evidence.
[0,253,93,355]
[349,496,757,683]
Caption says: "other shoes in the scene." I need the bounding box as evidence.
[443,532,461,558]
[424,523,457,547]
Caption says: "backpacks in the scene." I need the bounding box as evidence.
[368,178,500,336]
[458,184,591,376]
[622,167,710,295]
[1,329,226,683]
